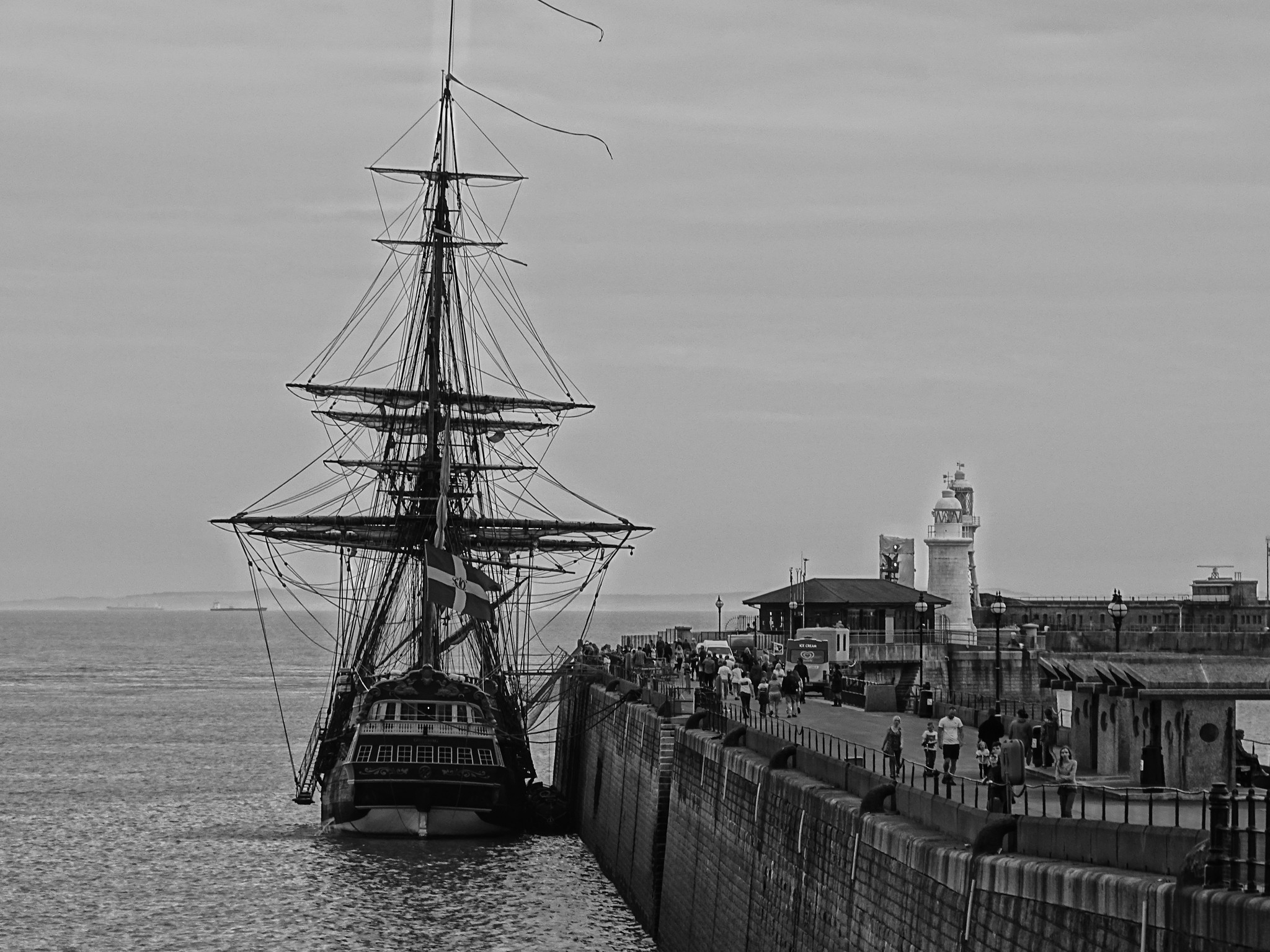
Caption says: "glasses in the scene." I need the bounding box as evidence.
[797,660,800,662]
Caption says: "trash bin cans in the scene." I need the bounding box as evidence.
[917,690,934,718]
[1138,745,1166,794]
[1031,726,1043,768]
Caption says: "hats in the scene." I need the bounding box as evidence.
[834,664,841,669]
[1018,709,1028,719]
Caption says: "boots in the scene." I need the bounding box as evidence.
[832,699,837,706]
[836,699,842,707]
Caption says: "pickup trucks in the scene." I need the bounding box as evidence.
[695,639,736,665]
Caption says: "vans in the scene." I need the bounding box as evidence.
[783,640,830,698]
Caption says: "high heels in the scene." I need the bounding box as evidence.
[690,678,694,681]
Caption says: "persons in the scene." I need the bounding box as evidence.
[879,707,1078,819]
[581,618,844,717]
[1235,729,1270,777]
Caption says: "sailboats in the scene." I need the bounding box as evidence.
[212,0,654,845]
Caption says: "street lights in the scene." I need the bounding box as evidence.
[1107,587,1128,653]
[915,591,927,687]
[715,594,723,640]
[991,591,1006,713]
[789,597,798,640]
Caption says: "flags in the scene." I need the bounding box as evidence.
[422,540,502,623]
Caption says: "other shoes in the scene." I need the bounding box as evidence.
[672,667,684,673]
[942,776,946,784]
[787,716,791,718]
[792,714,797,717]
[800,701,805,704]
[743,715,749,718]
[769,710,773,717]
[925,773,933,777]
[1260,768,1269,777]
[721,691,740,700]
[797,707,801,714]
[696,679,699,681]
[1243,783,1255,788]
[980,774,987,779]
[775,715,779,718]
[950,780,956,785]
[761,716,766,718]
[1052,763,1057,767]
[1040,765,1046,769]
[890,774,900,778]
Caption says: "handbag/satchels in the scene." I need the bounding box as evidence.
[884,750,890,757]
[986,800,1003,813]
